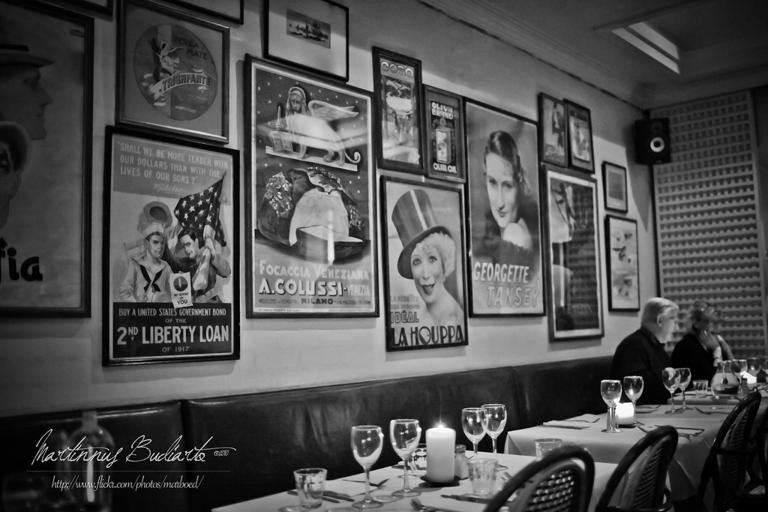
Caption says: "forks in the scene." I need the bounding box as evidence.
[693,407,729,416]
[343,478,389,489]
[409,499,451,512]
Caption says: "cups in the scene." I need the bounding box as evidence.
[534,439,561,461]
[692,380,708,398]
[290,467,327,512]
[466,458,497,497]
[411,443,427,472]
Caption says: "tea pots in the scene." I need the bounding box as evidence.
[709,360,741,400]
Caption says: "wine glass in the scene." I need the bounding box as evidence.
[389,419,420,498]
[623,376,643,428]
[676,369,691,412]
[350,425,383,510]
[661,368,680,414]
[599,380,622,433]
[481,404,508,473]
[461,407,489,458]
[733,357,761,392]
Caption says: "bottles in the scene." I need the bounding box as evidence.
[454,445,468,480]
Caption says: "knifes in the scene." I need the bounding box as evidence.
[440,494,490,505]
[287,488,354,504]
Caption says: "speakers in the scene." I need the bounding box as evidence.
[634,118,670,164]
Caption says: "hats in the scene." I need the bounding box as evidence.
[0,39,55,68]
[391,189,453,278]
[145,201,172,226]
[1,121,29,169]
[143,223,165,239]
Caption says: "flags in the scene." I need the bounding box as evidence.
[174,172,228,255]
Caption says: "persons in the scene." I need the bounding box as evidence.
[176,232,232,302]
[388,191,463,331]
[121,222,177,307]
[667,301,738,386]
[611,293,682,407]
[474,128,537,283]
